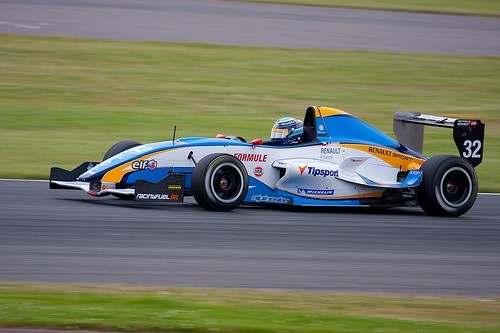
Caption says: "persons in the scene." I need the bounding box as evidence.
[216,117,304,146]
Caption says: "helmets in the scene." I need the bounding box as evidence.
[270,116,304,145]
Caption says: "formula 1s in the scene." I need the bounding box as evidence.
[50,103,486,218]
